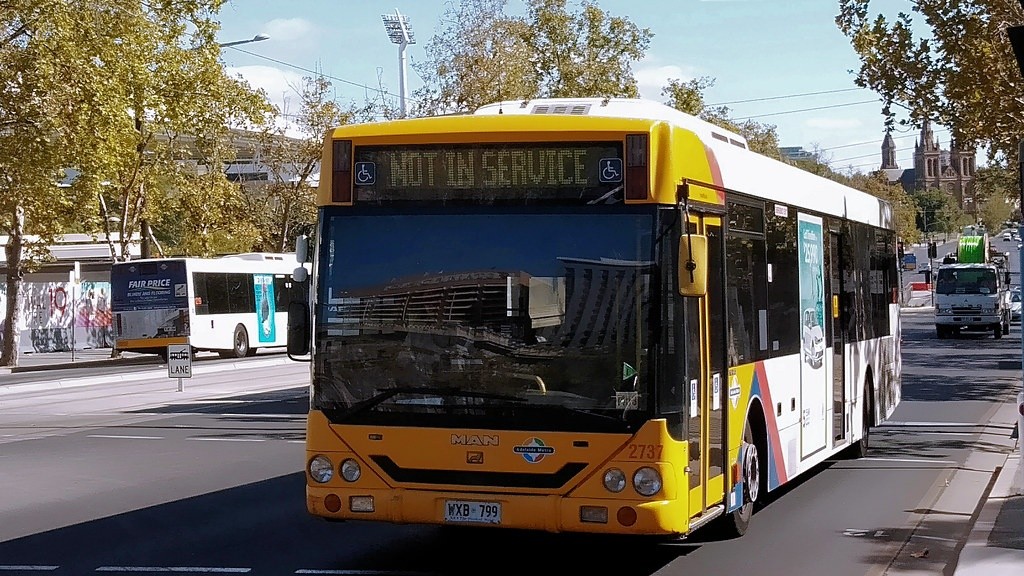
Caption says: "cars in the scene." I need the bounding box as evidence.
[1004,228,1022,251]
[1010,290,1023,319]
[919,262,931,274]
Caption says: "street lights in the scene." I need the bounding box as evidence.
[381,6,413,118]
[131,29,269,258]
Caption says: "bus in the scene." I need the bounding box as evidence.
[287,96,916,536]
[105,252,314,360]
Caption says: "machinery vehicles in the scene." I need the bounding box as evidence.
[934,233,1014,339]
